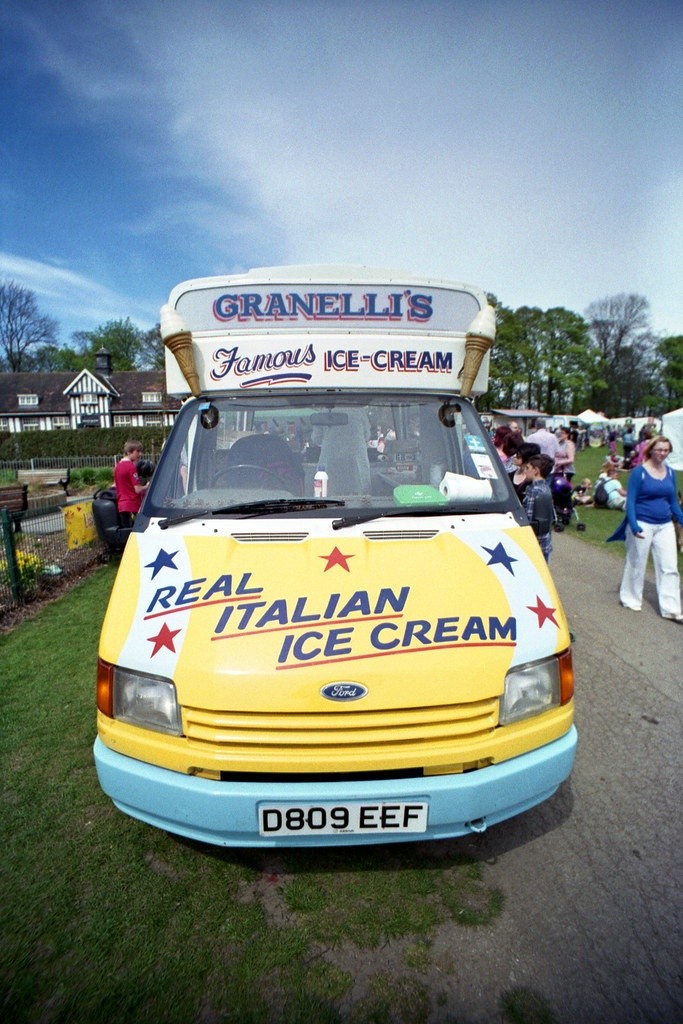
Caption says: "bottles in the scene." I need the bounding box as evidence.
[313,465,329,497]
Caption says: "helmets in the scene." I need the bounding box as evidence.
[136,460,155,478]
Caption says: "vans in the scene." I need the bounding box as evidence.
[91,263,581,850]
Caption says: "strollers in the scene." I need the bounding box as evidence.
[548,465,585,531]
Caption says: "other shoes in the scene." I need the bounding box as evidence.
[585,501,593,505]
[577,502,583,506]
[662,613,683,621]
[628,605,642,611]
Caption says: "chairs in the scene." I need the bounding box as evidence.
[226,434,305,497]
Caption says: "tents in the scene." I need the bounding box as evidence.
[577,408,609,424]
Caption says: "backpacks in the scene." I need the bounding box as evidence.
[594,477,617,508]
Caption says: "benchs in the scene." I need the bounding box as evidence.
[16,469,70,497]
[0,486,29,534]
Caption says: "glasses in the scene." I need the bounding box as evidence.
[652,448,670,453]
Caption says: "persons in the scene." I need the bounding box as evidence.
[607,436,683,622]
[485,421,654,565]
[113,439,151,527]
[161,439,188,496]
[231,418,312,452]
